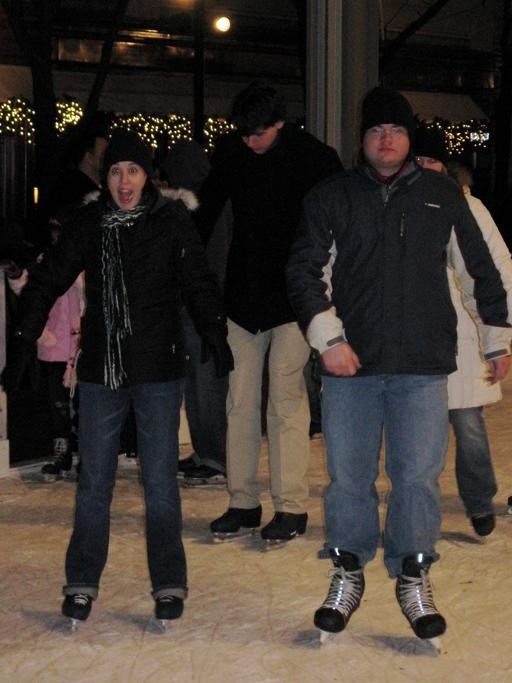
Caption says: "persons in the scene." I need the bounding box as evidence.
[2,126,113,481]
[199,83,346,536]
[1,132,230,621]
[410,125,512,535]
[283,88,512,639]
[150,140,230,484]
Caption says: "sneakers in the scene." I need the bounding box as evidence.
[210,505,261,533]
[180,453,221,479]
[314,547,364,632]
[261,511,308,539]
[155,594,184,619]
[62,594,92,621]
[396,554,445,639]
[471,513,494,535]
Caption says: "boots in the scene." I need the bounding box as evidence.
[41,436,80,475]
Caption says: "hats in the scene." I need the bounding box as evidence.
[411,127,448,166]
[99,131,154,174]
[360,85,414,144]
[231,77,288,132]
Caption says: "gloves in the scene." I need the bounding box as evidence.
[199,327,234,379]
[1,329,37,399]
